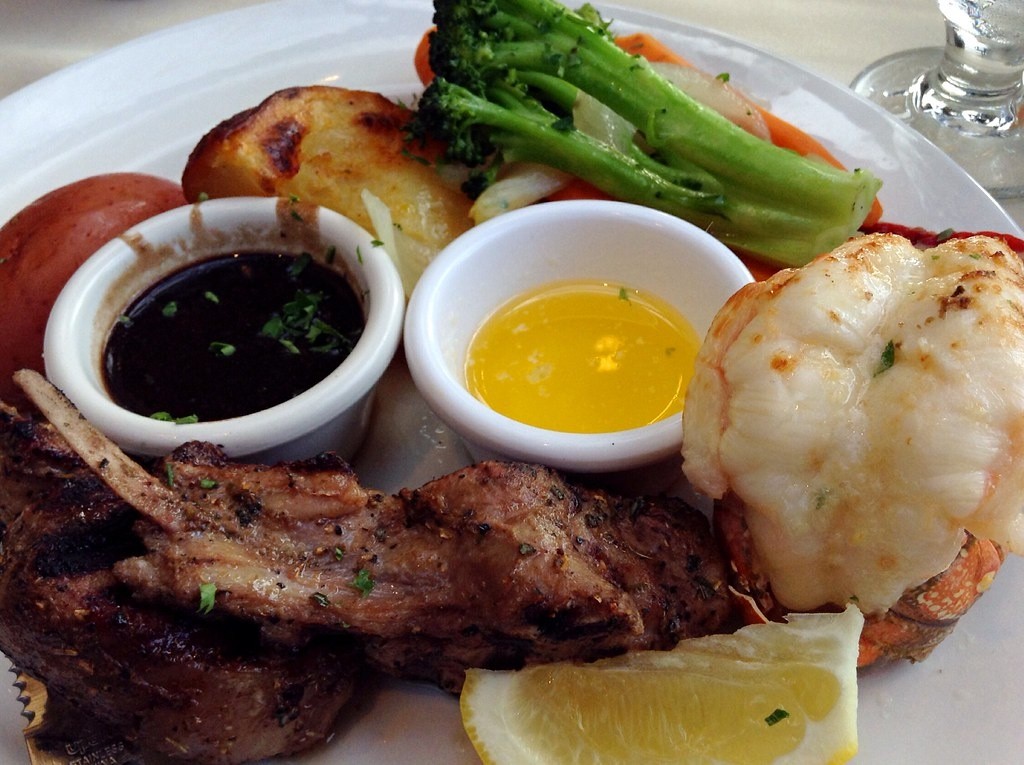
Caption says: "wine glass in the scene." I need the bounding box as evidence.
[849,0,1024,200]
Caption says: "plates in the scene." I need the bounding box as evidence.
[0,0,1024,765]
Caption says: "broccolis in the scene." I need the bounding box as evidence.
[411,1,884,263]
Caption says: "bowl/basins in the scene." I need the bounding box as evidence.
[44,196,406,467]
[404,199,757,472]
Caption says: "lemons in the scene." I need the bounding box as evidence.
[461,610,861,765]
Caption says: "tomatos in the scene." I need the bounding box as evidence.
[0,175,181,409]
